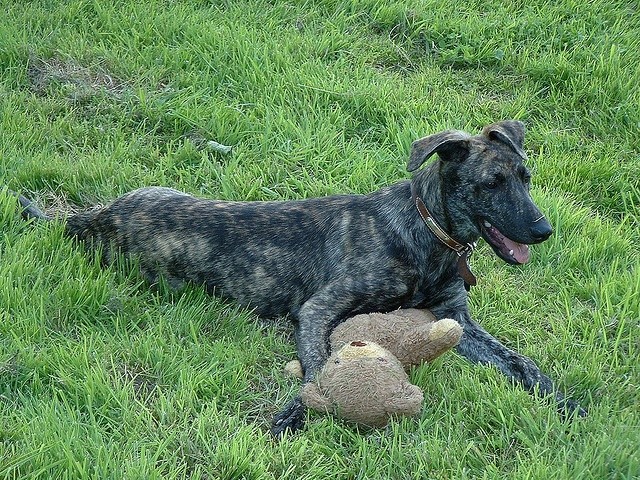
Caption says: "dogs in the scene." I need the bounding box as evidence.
[12,119,607,437]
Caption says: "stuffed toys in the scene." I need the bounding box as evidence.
[284,308,463,436]
